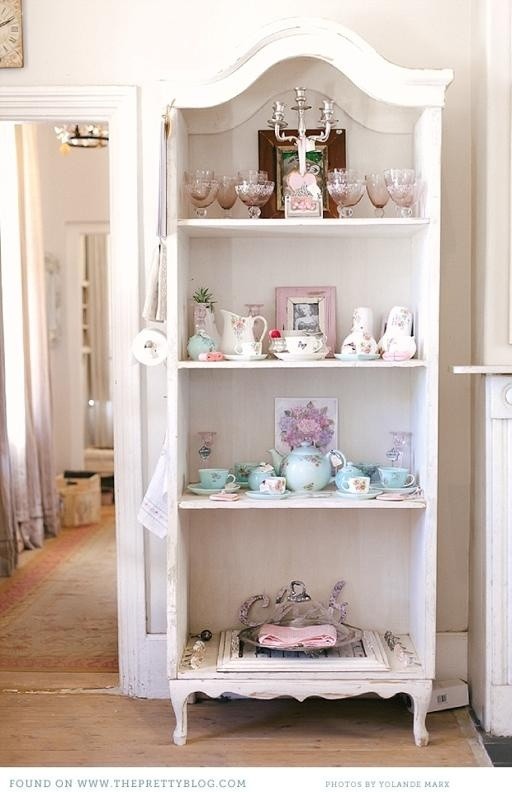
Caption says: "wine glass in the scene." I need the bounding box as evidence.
[183,168,417,218]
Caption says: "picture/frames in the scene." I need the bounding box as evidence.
[257,129,347,219]
[273,284,338,358]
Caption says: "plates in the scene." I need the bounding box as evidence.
[187,482,418,500]
[224,352,381,362]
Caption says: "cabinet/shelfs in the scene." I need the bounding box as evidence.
[144,24,456,744]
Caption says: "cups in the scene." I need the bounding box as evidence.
[197,461,415,495]
[234,306,416,360]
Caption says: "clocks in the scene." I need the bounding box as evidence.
[0,0,24,68]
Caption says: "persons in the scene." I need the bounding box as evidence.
[295,306,321,337]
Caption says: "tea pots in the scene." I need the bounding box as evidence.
[266,441,347,491]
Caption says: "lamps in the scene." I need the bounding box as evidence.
[54,122,110,156]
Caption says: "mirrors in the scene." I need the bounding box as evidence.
[67,217,113,473]
